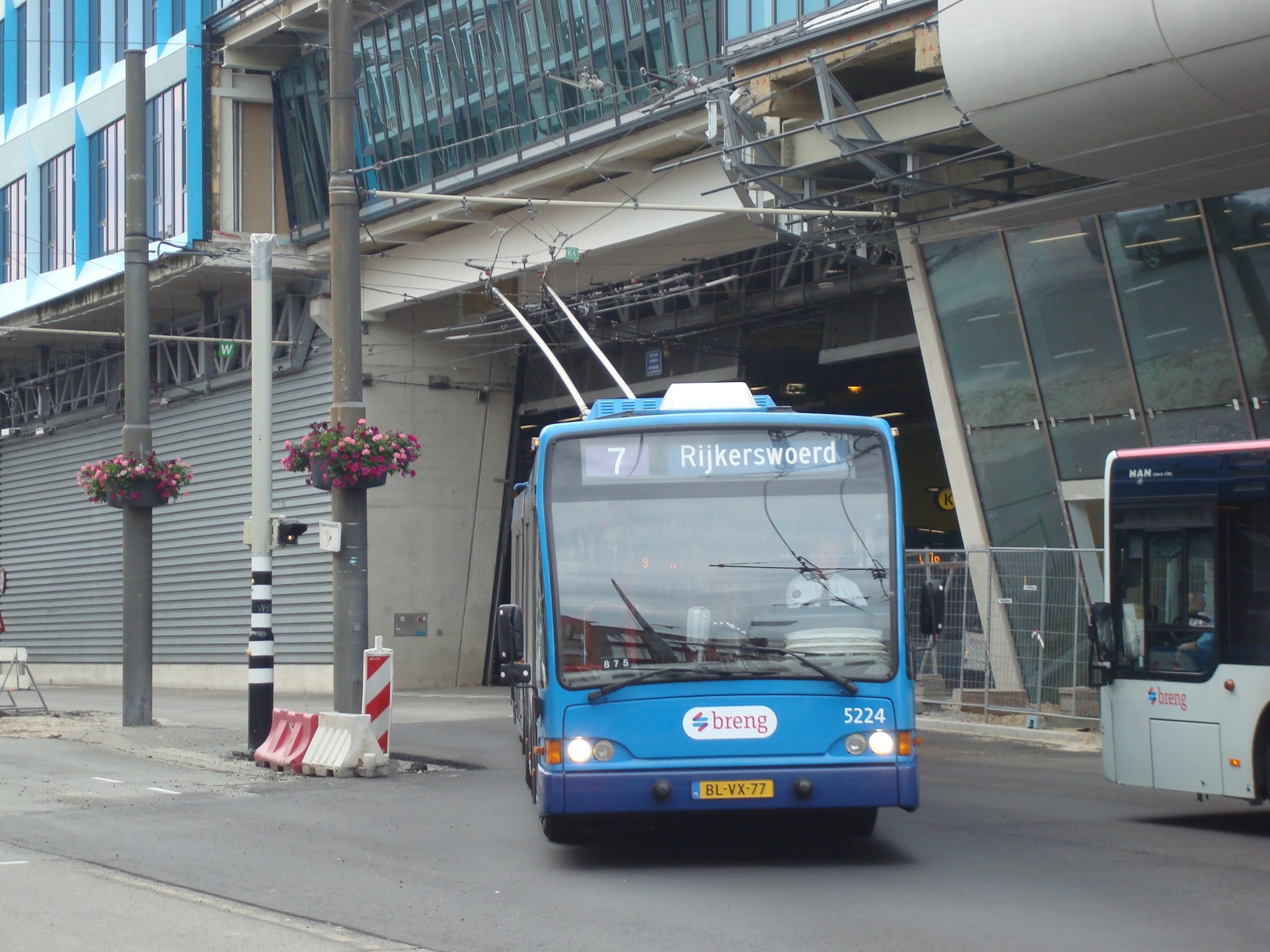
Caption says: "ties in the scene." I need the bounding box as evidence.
[819,581,831,607]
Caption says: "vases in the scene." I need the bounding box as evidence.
[305,454,386,493]
[107,479,170,509]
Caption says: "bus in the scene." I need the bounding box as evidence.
[1084,435,1270,803]
[497,382,948,842]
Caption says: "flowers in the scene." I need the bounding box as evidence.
[279,416,421,489]
[78,456,195,505]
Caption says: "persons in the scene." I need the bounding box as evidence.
[786,540,868,610]
[1176,615,1218,673]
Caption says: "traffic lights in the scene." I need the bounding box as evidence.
[277,519,308,550]
